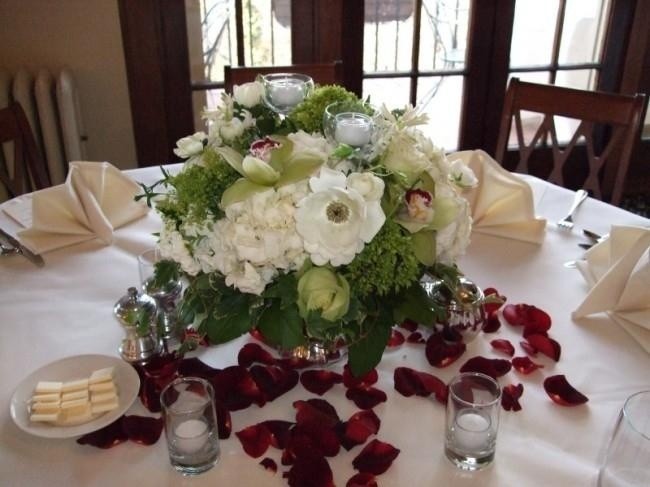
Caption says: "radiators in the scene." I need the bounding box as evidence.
[0,62,90,188]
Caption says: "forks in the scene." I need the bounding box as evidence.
[555,186,589,231]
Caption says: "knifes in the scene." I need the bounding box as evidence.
[0,230,46,269]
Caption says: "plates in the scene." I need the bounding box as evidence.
[9,354,141,440]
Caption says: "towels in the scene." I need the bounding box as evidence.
[433,149,546,247]
[572,226,650,355]
[15,160,148,257]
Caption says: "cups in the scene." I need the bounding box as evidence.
[598,390,650,487]
[159,375,220,473]
[444,372,501,473]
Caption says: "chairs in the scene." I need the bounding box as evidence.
[2,102,51,206]
[492,77,646,208]
[223,60,341,99]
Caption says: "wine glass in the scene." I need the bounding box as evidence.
[322,100,387,163]
[137,249,186,342]
[262,71,317,122]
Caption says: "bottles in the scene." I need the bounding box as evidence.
[112,285,159,363]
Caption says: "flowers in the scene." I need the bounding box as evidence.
[133,72,477,382]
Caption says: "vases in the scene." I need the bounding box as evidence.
[282,335,336,364]
